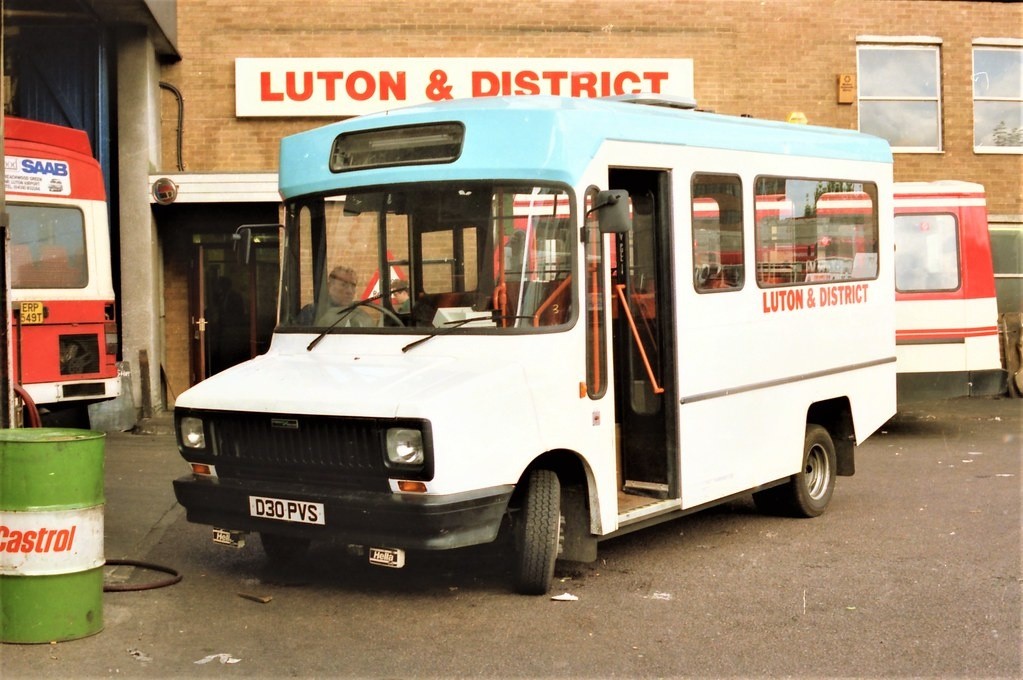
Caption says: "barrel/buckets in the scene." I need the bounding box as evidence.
[0,427,108,645]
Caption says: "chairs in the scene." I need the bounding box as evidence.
[414,254,852,336]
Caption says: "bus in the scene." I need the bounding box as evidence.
[2,117,123,428]
[484,180,1011,401]
[174,95,896,596]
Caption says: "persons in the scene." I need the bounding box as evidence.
[392,279,410,312]
[217,276,246,326]
[296,266,373,327]
